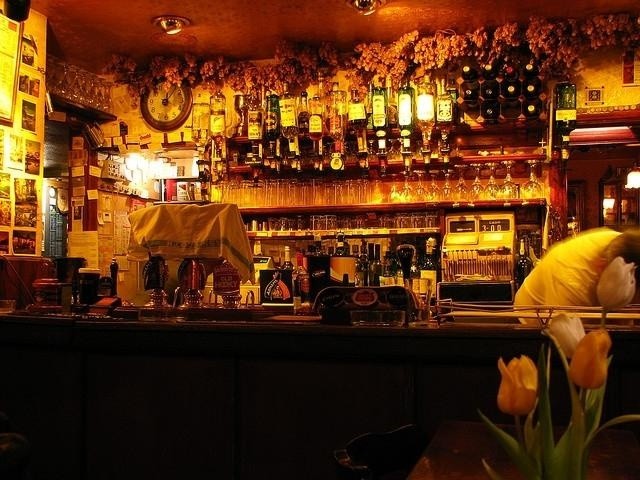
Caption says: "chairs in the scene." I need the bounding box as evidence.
[334,422,428,479]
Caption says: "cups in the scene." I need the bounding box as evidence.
[409,276,434,323]
[244,211,439,231]
[166,178,385,207]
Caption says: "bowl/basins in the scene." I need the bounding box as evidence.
[348,310,407,328]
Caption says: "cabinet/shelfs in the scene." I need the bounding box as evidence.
[198,146,568,297]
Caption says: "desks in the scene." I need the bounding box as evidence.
[404,419,639,480]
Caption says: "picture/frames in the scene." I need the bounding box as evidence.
[0,13,24,126]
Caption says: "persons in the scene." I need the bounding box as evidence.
[515,227,640,326]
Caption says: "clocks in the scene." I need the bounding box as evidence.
[140,78,193,131]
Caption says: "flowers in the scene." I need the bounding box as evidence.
[472,257,640,480]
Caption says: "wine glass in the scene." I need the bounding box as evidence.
[387,159,545,204]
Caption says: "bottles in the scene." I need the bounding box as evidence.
[193,63,576,182]
[514,236,533,292]
[247,233,440,302]
